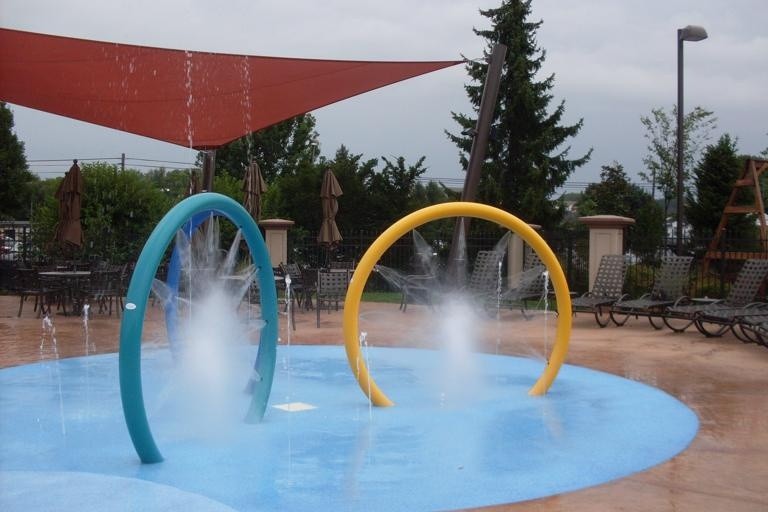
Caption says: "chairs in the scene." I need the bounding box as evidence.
[11,237,365,332]
[391,237,767,347]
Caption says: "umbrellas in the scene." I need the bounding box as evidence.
[179,170,211,248]
[239,161,270,271]
[52,158,88,275]
[314,165,345,269]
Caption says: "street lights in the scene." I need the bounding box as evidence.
[674,25,708,250]
